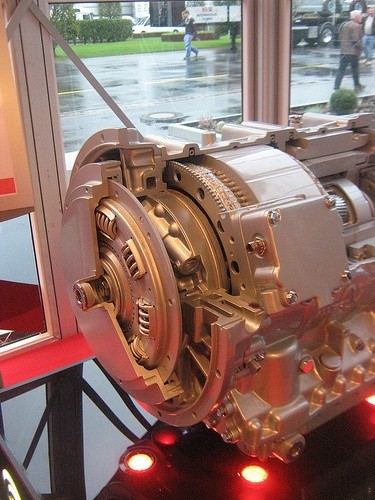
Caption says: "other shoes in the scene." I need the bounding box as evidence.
[195,50,199,57]
[183,56,191,60]
[360,58,375,65]
[354,84,367,91]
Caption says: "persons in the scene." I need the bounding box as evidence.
[183,10,199,59]
[333,10,368,92]
[361,8,375,65]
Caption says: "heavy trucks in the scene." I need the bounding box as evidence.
[291,1,372,45]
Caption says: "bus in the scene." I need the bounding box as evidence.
[130,15,187,34]
